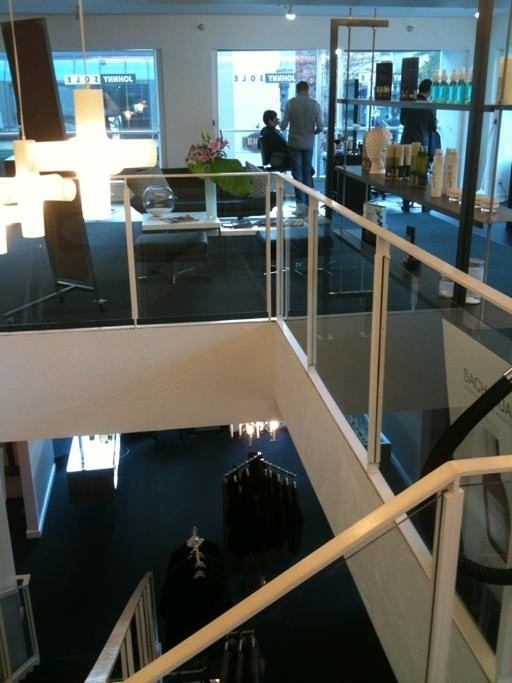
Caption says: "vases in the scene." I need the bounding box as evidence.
[203,177,219,219]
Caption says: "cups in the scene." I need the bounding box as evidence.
[439,258,484,304]
[449,191,500,212]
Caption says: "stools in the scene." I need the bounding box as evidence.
[257,224,333,281]
[131,232,212,285]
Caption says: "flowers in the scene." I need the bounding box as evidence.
[184,117,254,198]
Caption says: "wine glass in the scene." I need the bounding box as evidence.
[334,134,341,150]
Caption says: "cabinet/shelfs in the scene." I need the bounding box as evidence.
[320,2,512,345]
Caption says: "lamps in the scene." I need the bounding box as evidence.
[0,0,159,259]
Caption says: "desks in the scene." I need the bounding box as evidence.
[322,152,370,214]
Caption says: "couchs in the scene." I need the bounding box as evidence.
[125,164,282,218]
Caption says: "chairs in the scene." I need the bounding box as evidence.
[256,136,288,171]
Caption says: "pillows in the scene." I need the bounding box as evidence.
[243,159,281,198]
[126,162,178,200]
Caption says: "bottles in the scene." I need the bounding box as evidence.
[416,145,459,198]
[221,631,262,683]
[431,67,475,103]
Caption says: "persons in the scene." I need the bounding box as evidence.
[278,81,323,217]
[260,110,316,176]
[399,79,436,213]
